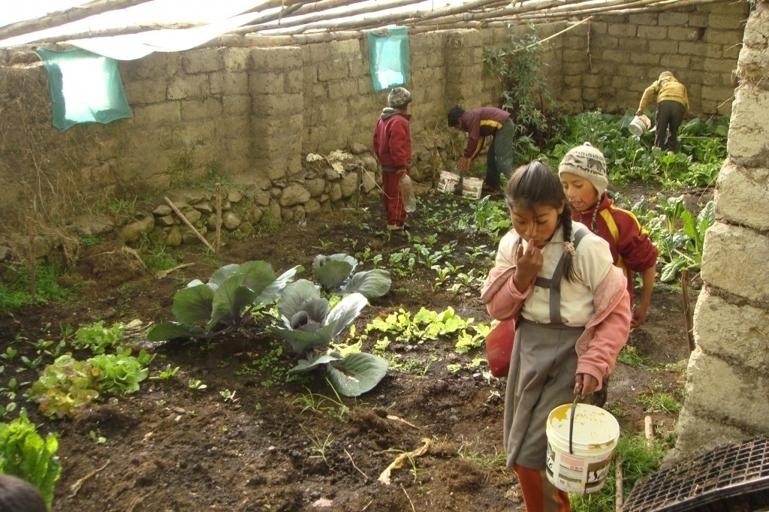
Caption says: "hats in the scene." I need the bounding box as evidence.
[558,144,608,197]
[387,87,412,108]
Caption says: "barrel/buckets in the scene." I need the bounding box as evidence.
[462,177,485,199]
[437,169,460,194]
[546,396,620,495]
[628,114,652,136]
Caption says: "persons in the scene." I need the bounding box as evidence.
[555,140,661,408]
[447,104,513,197]
[372,86,416,240]
[479,156,632,511]
[636,69,690,153]
